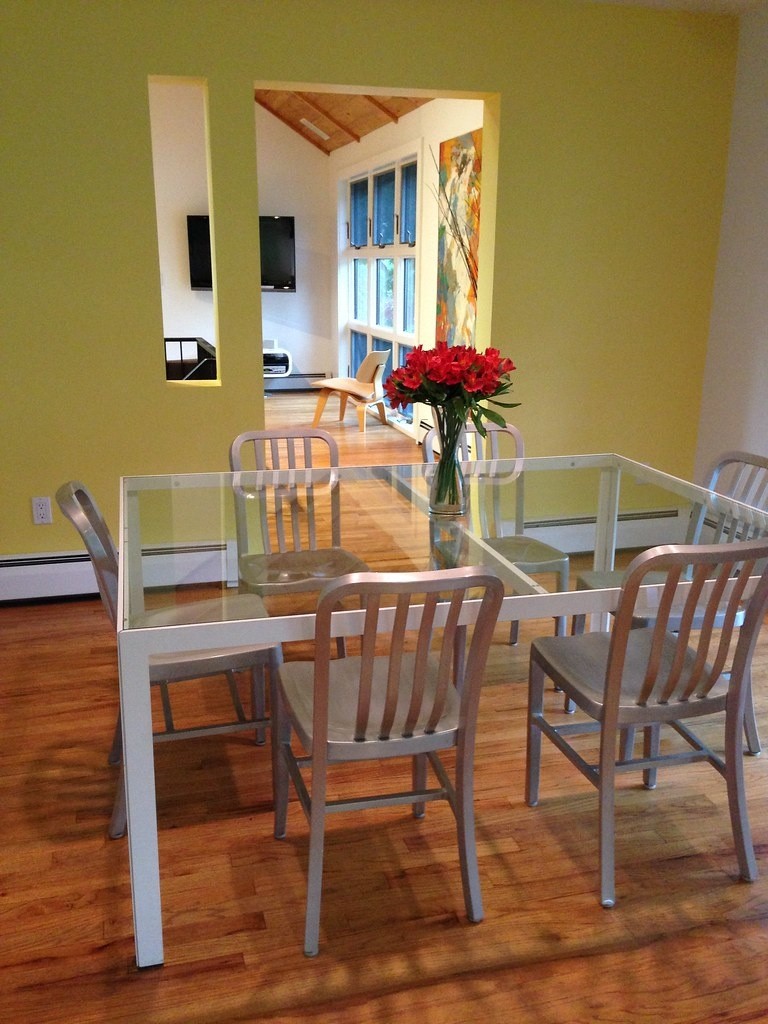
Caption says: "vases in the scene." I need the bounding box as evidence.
[429,406,466,514]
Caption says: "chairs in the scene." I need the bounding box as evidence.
[228,430,373,723]
[522,538,768,911]
[309,347,391,433]
[568,450,768,760]
[275,566,507,957]
[420,423,574,695]
[53,480,291,845]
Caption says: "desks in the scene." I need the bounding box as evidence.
[115,451,768,968]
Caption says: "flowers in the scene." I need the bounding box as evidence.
[383,340,522,507]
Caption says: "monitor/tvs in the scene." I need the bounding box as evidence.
[186,214,296,293]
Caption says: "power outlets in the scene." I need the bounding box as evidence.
[30,495,56,527]
